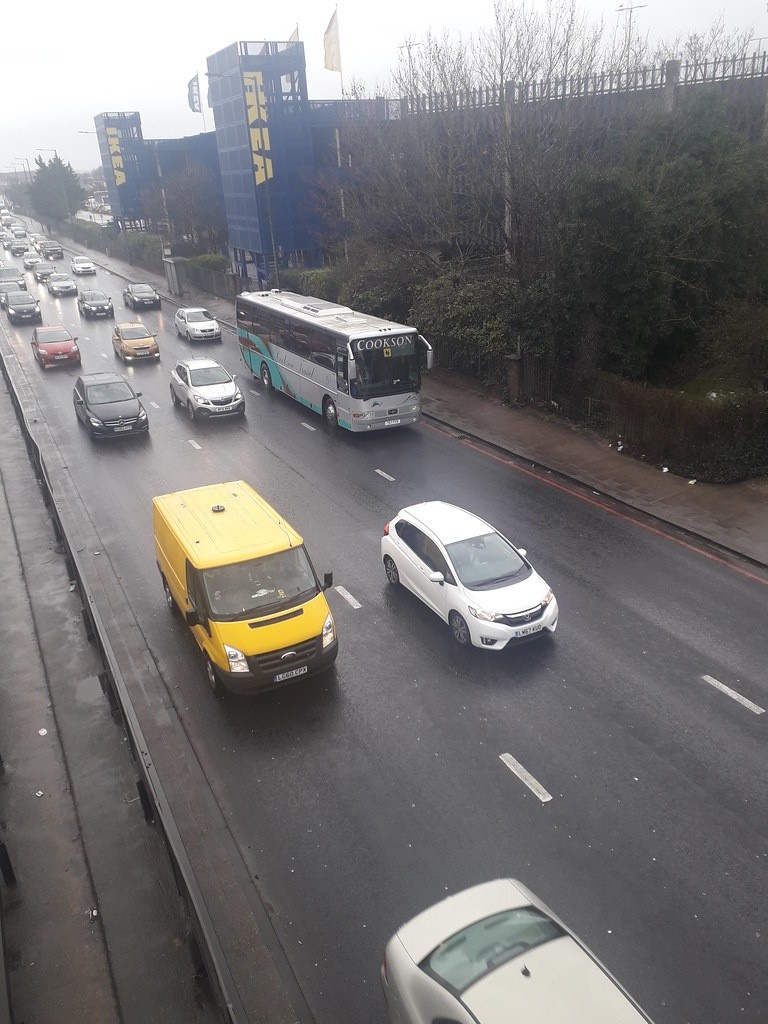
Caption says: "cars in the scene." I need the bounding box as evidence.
[0,202,65,291]
[70,255,97,277]
[123,282,162,310]
[174,306,223,342]
[2,291,43,325]
[379,876,655,1024]
[112,322,161,363]
[73,371,149,439]
[30,325,81,368]
[33,263,58,282]
[77,289,115,319]
[47,273,79,297]
[0,282,23,309]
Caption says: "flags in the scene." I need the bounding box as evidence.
[258,28,299,83]
[187,75,201,112]
[323,10,342,72]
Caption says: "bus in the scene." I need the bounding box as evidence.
[235,288,435,433]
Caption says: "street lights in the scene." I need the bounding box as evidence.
[202,70,280,290]
[76,129,133,268]
[35,148,70,211]
[14,157,34,183]
[11,162,28,183]
[4,166,19,183]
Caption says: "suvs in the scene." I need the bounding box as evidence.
[380,501,559,652]
[169,356,246,420]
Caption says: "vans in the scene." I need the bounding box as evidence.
[152,480,340,700]
